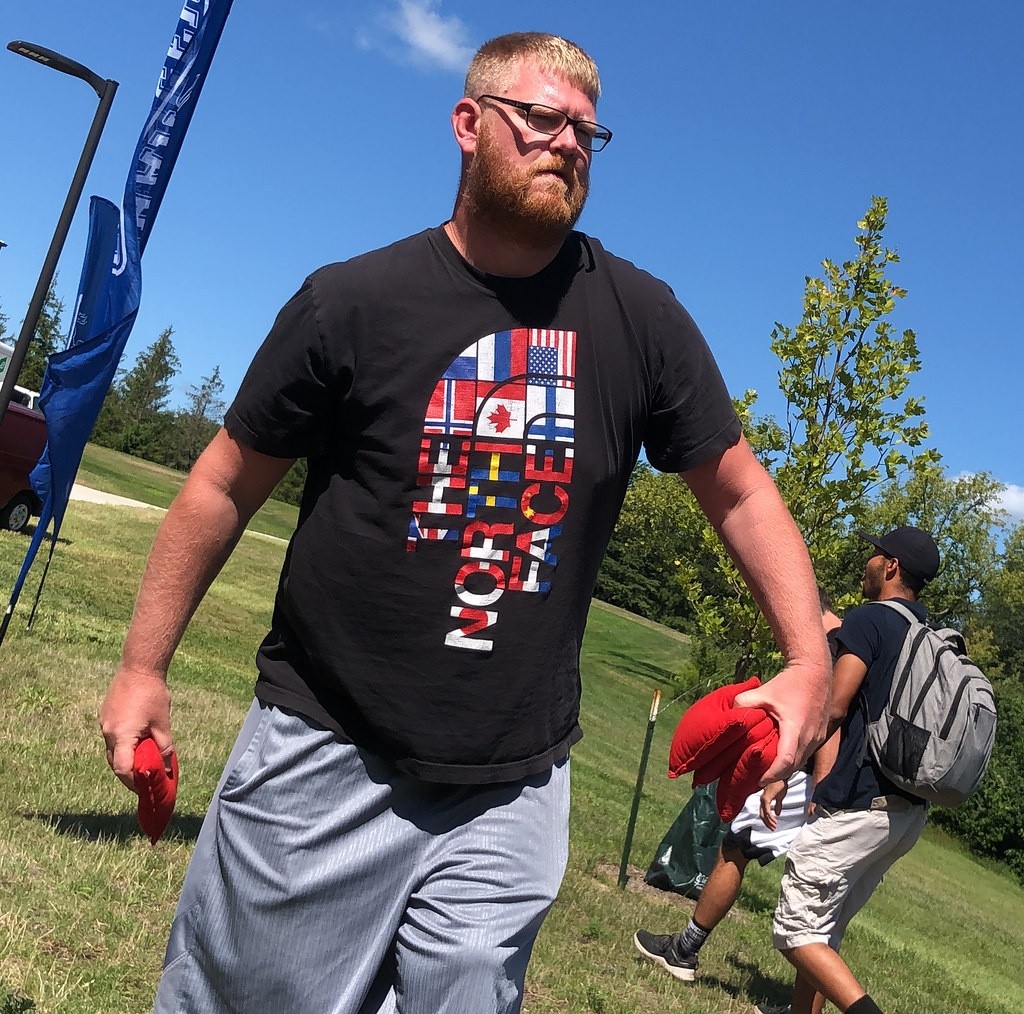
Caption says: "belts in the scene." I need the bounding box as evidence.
[879,787,927,805]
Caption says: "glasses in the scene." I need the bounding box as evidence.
[476,94,613,152]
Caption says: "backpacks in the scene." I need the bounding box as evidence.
[855,599,996,808]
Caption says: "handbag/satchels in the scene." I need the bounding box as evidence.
[643,777,730,902]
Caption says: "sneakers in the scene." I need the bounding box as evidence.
[754,1004,792,1014]
[633,928,699,981]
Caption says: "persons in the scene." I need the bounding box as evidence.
[634,527,940,1014]
[103,32,832,1013]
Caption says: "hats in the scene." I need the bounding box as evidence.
[855,525,940,586]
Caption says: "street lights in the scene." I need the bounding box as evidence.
[0,37,120,411]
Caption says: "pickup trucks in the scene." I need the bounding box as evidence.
[1,342,45,531]
